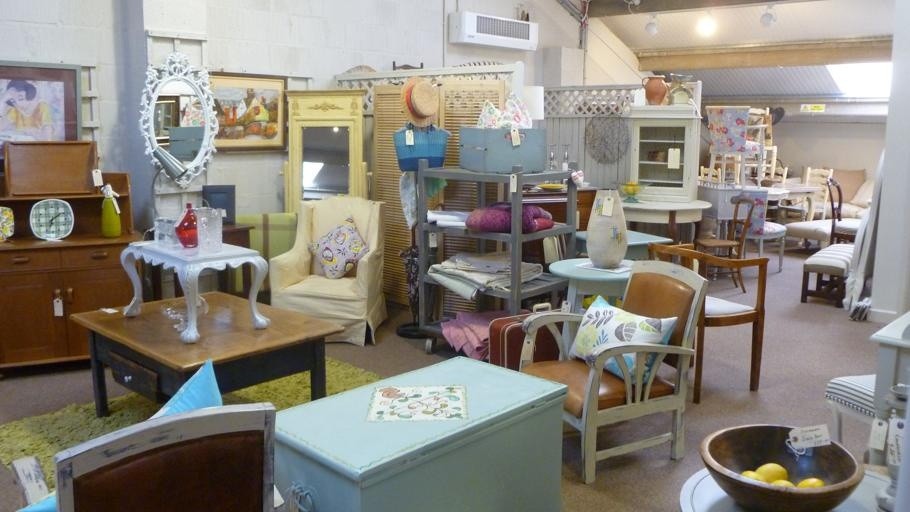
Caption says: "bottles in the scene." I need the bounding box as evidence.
[546,143,568,184]
[100,183,122,238]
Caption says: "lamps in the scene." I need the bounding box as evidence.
[149,147,187,217]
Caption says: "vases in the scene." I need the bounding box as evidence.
[175,203,197,250]
[641,76,668,105]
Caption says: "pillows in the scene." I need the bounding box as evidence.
[17,359,282,512]
[307,216,365,280]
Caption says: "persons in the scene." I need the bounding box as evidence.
[0,79,58,143]
[232,87,270,135]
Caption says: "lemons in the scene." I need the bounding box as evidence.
[623,182,640,194]
[771,479,796,488]
[741,470,761,481]
[756,462,788,485]
[797,478,824,489]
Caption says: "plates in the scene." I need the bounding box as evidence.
[0,206,16,240]
[537,185,568,191]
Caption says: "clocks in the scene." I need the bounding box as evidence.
[30,199,76,240]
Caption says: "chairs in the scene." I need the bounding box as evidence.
[517,103,910,512]
[13,399,295,512]
[268,197,388,348]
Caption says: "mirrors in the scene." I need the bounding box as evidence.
[139,53,220,188]
[284,91,368,212]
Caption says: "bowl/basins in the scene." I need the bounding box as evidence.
[695,423,864,508]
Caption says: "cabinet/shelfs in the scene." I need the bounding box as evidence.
[417,162,576,354]
[0,176,144,376]
[373,78,509,318]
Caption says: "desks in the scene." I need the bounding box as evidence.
[122,237,270,343]
[68,291,346,416]
[273,357,572,512]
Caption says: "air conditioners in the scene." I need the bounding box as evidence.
[449,12,540,51]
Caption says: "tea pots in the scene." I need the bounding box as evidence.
[641,75,665,106]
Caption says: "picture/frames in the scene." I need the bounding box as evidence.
[1,61,83,162]
[209,72,287,152]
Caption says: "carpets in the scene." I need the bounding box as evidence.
[0,352,388,486]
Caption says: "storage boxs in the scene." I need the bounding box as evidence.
[460,127,549,172]
